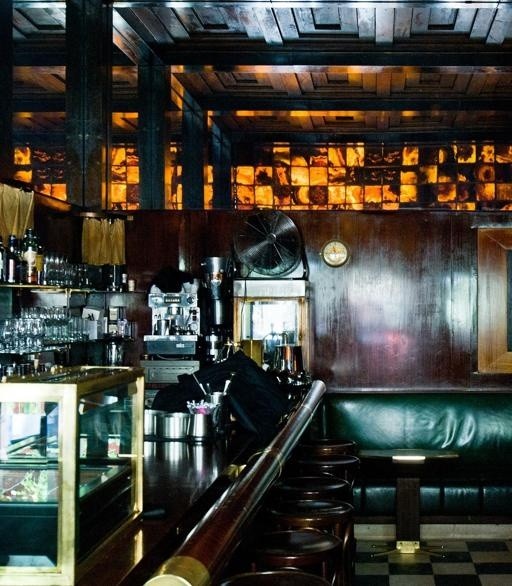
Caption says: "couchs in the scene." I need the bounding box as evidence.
[317,391,512,543]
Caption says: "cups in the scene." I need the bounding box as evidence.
[186,412,219,444]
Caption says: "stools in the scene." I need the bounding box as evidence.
[218,437,361,586]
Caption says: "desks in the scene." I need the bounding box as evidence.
[359,447,460,560]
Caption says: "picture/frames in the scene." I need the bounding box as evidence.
[477,226,512,375]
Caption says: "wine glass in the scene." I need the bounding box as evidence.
[118,319,127,335]
[0,318,42,348]
[25,305,84,340]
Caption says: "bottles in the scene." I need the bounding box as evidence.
[43,255,134,293]
[0,352,48,383]
[0,227,44,284]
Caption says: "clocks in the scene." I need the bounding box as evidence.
[321,239,350,267]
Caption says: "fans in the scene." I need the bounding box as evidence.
[225,207,310,280]
